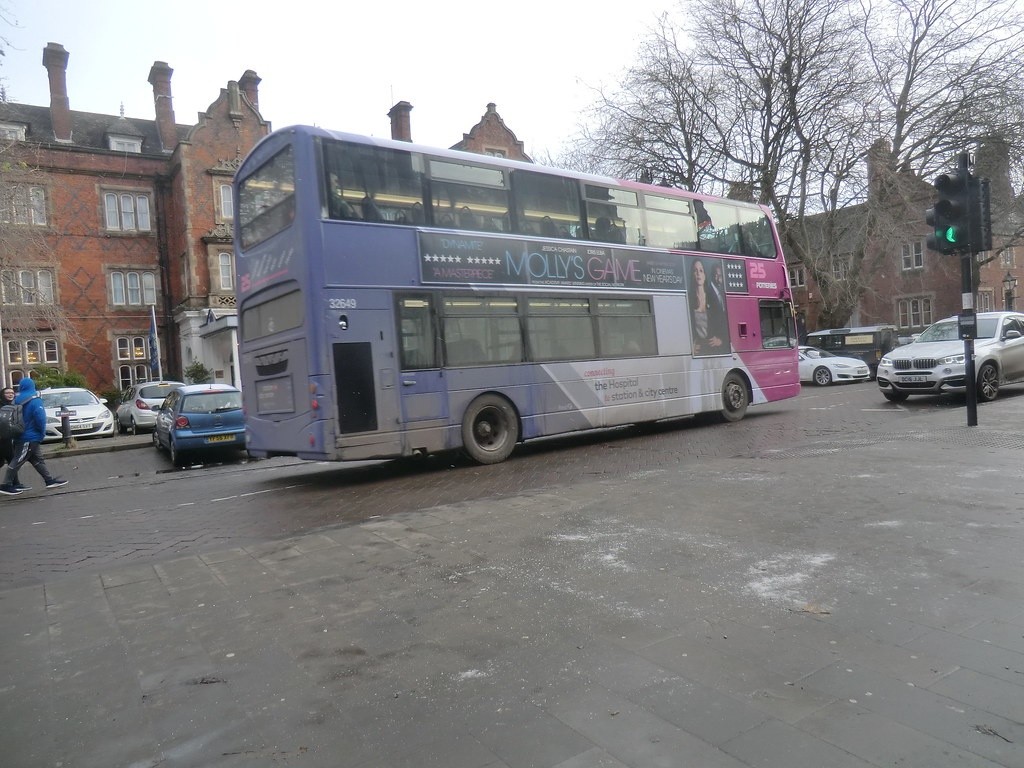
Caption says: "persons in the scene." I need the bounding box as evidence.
[329,172,358,220]
[0,387,33,491]
[0,377,70,496]
[687,258,731,356]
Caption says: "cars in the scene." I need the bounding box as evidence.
[876,310,1024,402]
[798,345,871,387]
[114,380,187,436]
[36,386,115,444]
[150,380,245,467]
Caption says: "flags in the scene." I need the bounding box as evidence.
[149,305,159,373]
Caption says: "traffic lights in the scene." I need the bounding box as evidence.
[922,205,951,255]
[931,169,971,254]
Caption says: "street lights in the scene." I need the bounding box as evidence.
[1001,270,1018,310]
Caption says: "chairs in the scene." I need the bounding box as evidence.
[402,338,639,364]
[327,196,625,245]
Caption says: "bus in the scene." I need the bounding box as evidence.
[231,126,801,465]
[804,325,899,373]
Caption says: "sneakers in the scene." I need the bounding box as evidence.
[0,484,23,495]
[46,480,68,488]
[14,484,32,491]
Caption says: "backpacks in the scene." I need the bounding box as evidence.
[0,396,40,437]
